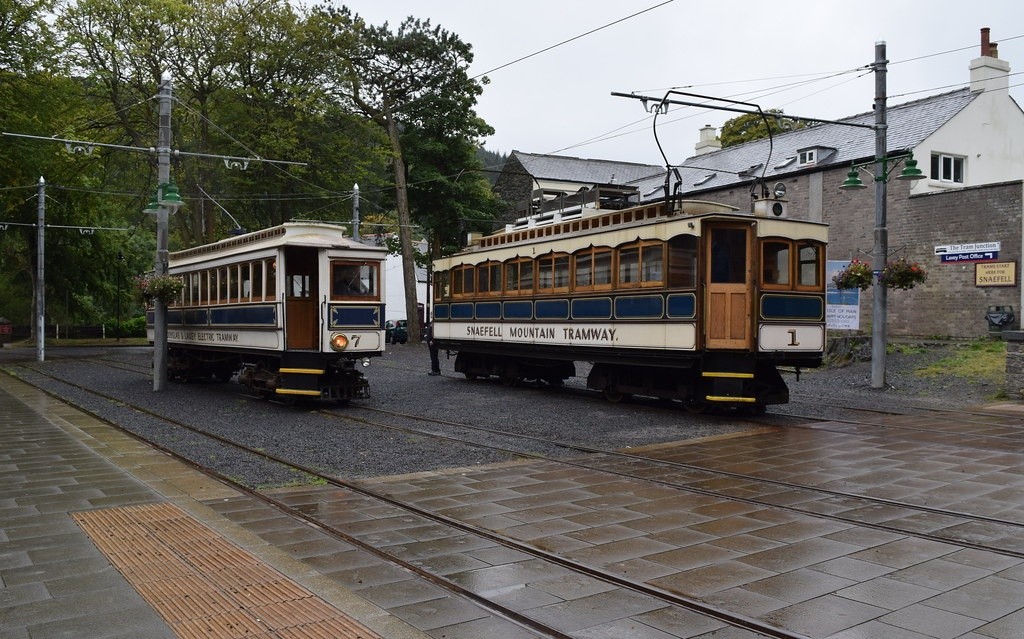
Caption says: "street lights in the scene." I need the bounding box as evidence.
[838,152,928,389]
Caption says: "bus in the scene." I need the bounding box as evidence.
[421,85,829,417]
[145,221,389,407]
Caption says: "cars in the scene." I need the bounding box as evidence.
[385,320,408,345]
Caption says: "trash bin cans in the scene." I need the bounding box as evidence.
[984,304,1016,337]
